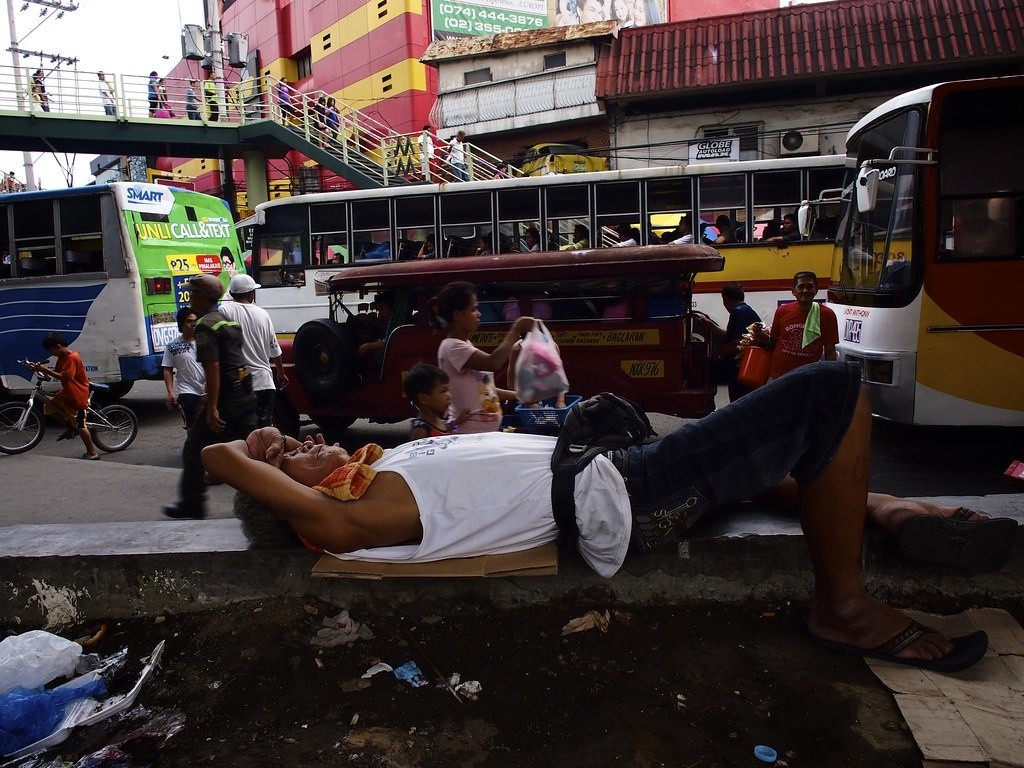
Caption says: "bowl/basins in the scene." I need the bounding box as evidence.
[0,639,165,760]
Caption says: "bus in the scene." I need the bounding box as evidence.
[798,75,1024,422]
[235,154,857,364]
[260,244,727,439]
[0,184,253,412]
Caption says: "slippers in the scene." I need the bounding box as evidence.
[901,506,1018,574]
[80,453,100,460]
[818,618,988,671]
[57,431,70,441]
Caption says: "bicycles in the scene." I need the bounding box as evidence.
[1,357,139,455]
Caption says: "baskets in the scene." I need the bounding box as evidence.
[514,395,582,427]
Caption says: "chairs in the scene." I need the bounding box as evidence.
[446,235,464,258]
[987,192,1015,251]
[397,242,411,260]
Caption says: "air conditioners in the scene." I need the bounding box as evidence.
[780,127,819,156]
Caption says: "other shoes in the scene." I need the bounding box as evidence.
[162,505,203,519]
[204,472,221,484]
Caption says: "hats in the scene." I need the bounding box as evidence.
[230,274,261,294]
[180,274,224,298]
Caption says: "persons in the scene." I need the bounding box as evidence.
[418,125,434,181]
[147,71,175,118]
[199,360,1024,676]
[700,280,764,404]
[328,222,639,264]
[0,172,27,193]
[554,0,661,28]
[30,70,50,112]
[161,273,288,520]
[314,97,340,150]
[186,78,202,120]
[447,131,470,182]
[274,77,295,127]
[665,212,801,246]
[758,272,840,384]
[493,162,507,179]
[32,332,101,460]
[97,71,116,115]
[403,281,567,440]
[204,72,219,121]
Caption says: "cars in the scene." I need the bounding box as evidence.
[515,142,611,180]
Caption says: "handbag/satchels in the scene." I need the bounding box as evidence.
[551,392,657,542]
[157,102,170,118]
[319,122,325,129]
[512,318,569,403]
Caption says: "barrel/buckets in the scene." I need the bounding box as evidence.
[738,345,771,388]
